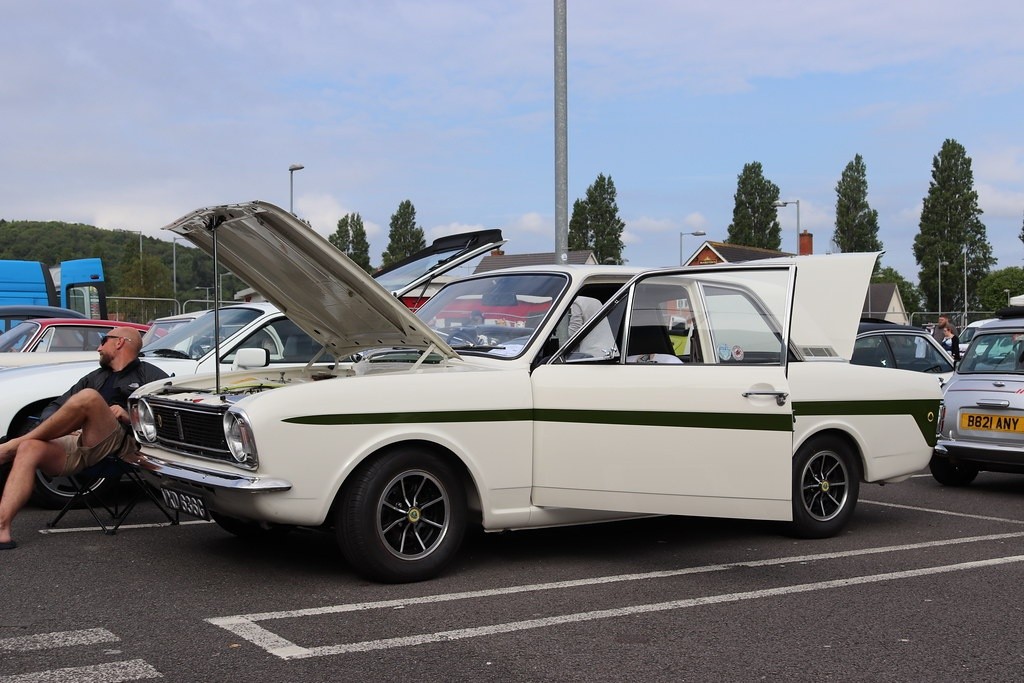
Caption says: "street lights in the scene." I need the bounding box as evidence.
[938,258,950,316]
[288,163,305,215]
[1003,289,1011,307]
[771,199,800,256]
[679,230,706,266]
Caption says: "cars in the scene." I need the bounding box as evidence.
[0,229,1024,511]
[929,317,1024,489]
[127,198,945,584]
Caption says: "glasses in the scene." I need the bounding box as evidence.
[101,335,131,346]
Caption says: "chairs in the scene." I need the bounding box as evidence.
[616,324,677,363]
[33,403,182,536]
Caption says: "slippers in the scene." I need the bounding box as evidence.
[0,540,17,550]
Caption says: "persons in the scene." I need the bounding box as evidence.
[914,337,927,359]
[0,327,170,550]
[931,315,958,346]
[942,324,961,362]
[568,296,620,359]
